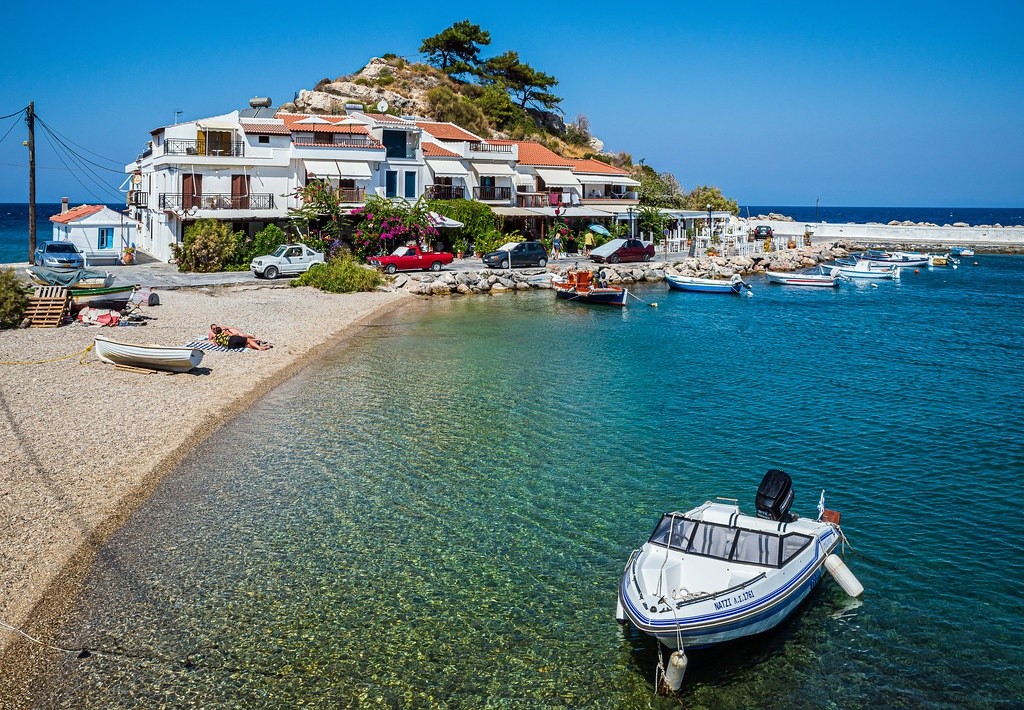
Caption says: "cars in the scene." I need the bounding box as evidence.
[589,238,654,264]
[249,245,327,279]
[33,240,85,269]
[754,226,774,240]
[483,241,549,268]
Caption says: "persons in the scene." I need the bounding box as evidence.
[585,229,596,260]
[208,324,270,351]
[591,189,596,196]
[552,232,563,260]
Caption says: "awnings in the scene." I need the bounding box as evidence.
[489,206,618,217]
[469,161,517,178]
[197,121,239,133]
[426,159,469,178]
[516,174,534,186]
[584,204,733,219]
[573,175,641,186]
[535,169,581,189]
[302,158,373,181]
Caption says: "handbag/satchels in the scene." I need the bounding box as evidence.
[551,247,556,255]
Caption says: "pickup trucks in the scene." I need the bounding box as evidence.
[366,245,454,273]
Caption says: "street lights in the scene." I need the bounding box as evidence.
[707,204,712,238]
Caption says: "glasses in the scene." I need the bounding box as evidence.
[212,328,216,330]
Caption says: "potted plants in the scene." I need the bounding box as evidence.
[123,248,134,265]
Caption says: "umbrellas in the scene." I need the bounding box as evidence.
[293,114,332,138]
[332,118,370,140]
[421,210,465,252]
[587,224,610,237]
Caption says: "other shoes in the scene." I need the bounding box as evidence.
[553,257,556,260]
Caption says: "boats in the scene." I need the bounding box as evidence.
[95,334,205,373]
[618,466,864,690]
[67,286,141,307]
[953,247,975,256]
[820,252,957,280]
[665,273,752,295]
[549,267,629,306]
[766,269,849,286]
[23,267,116,288]
